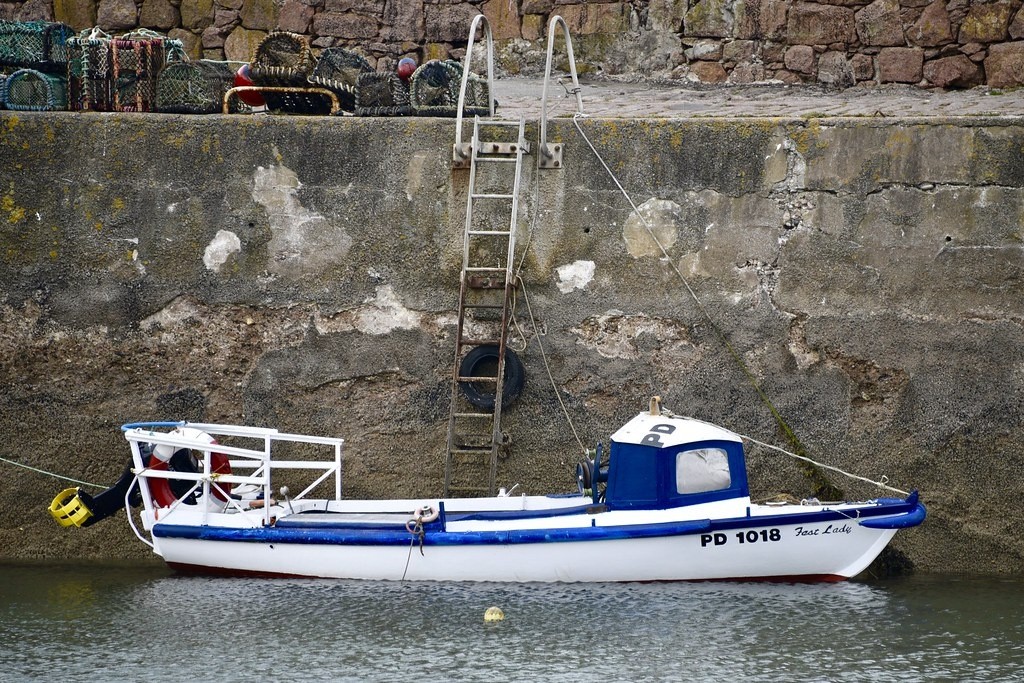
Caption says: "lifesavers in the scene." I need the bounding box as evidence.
[148,429,232,513]
[458,344,524,409]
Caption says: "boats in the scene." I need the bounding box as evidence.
[44,393,930,585]
